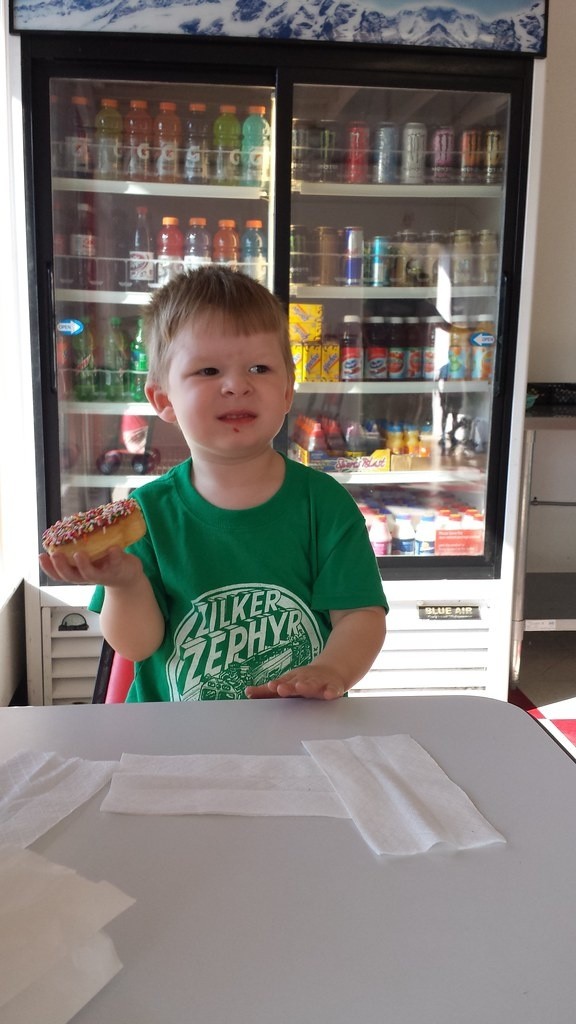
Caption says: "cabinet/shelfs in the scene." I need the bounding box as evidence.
[513,415,576,685]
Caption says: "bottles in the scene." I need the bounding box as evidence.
[49,95,152,182]
[152,101,271,187]
[390,228,500,287]
[153,216,268,292]
[131,447,161,475]
[352,490,485,556]
[293,412,383,452]
[52,198,157,293]
[341,315,496,382]
[56,315,149,404]
[97,449,124,474]
[376,418,432,457]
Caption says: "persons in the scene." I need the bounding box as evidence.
[40,268,389,702]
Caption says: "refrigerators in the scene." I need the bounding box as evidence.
[5,0,550,703]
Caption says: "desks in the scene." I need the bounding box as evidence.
[0,693,576,1024]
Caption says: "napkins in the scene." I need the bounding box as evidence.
[302,733,506,858]
[0,848,137,1024]
[102,754,350,821]
[0,752,119,849]
